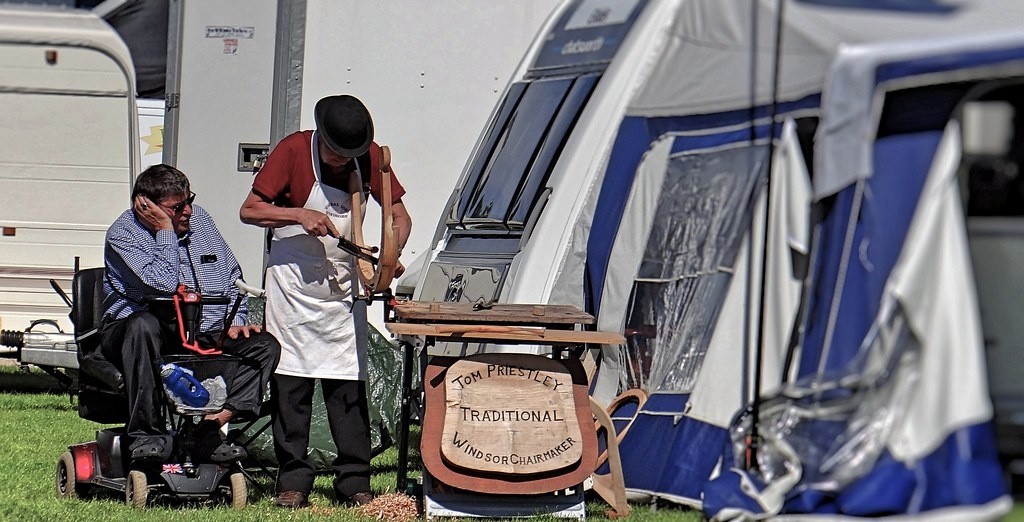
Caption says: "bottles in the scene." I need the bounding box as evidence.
[160,364,210,408]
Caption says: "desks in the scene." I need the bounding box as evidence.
[383,300,626,493]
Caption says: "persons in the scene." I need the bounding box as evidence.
[240,95,412,507]
[102,163,282,462]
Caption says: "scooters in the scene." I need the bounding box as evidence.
[56,267,267,511]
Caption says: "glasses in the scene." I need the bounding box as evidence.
[171,191,196,212]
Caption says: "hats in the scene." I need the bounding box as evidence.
[314,94,374,158]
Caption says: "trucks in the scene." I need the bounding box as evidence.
[0,0,140,372]
[393,0,1024,427]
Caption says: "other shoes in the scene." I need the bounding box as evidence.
[347,492,373,505]
[276,490,308,508]
[193,441,247,461]
[131,437,165,457]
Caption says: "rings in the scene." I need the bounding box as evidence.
[142,203,147,208]
